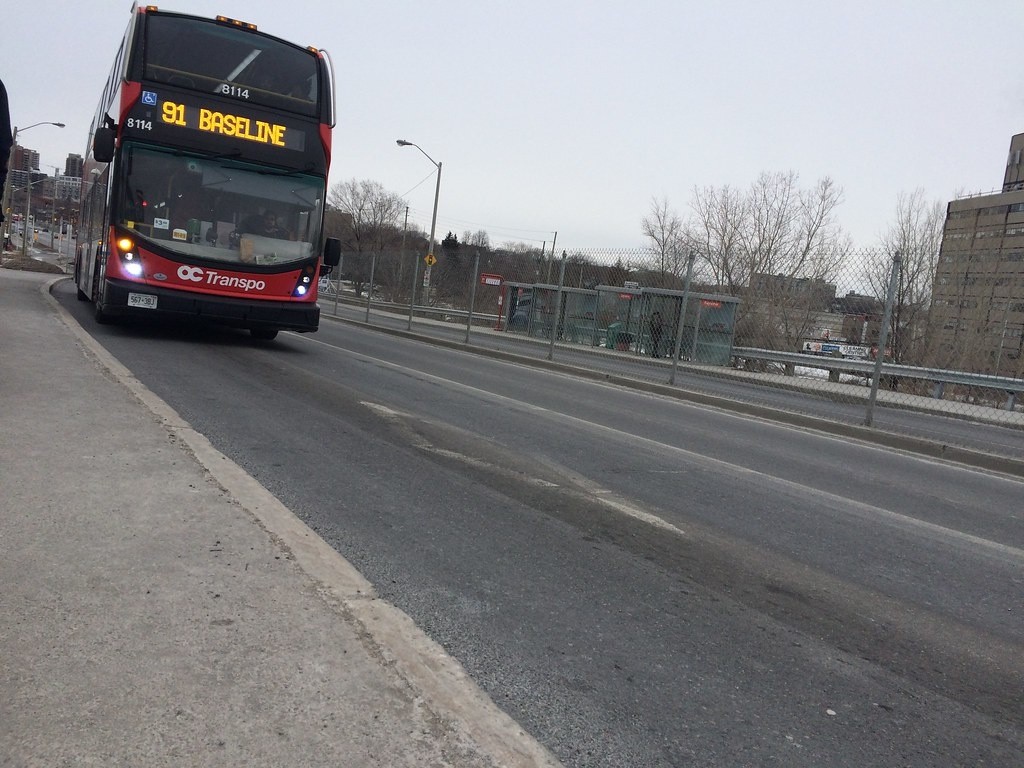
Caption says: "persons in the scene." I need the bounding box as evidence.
[243,209,289,241]
[649,311,662,358]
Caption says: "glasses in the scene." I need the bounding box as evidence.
[265,217,275,221]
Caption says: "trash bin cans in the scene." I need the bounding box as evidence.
[606,322,622,349]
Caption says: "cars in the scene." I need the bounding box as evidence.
[42,228,49,232]
[54,233,59,239]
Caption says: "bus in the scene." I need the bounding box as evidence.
[75,1,342,345]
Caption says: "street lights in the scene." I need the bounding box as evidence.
[0,122,65,264]
[395,139,442,305]
